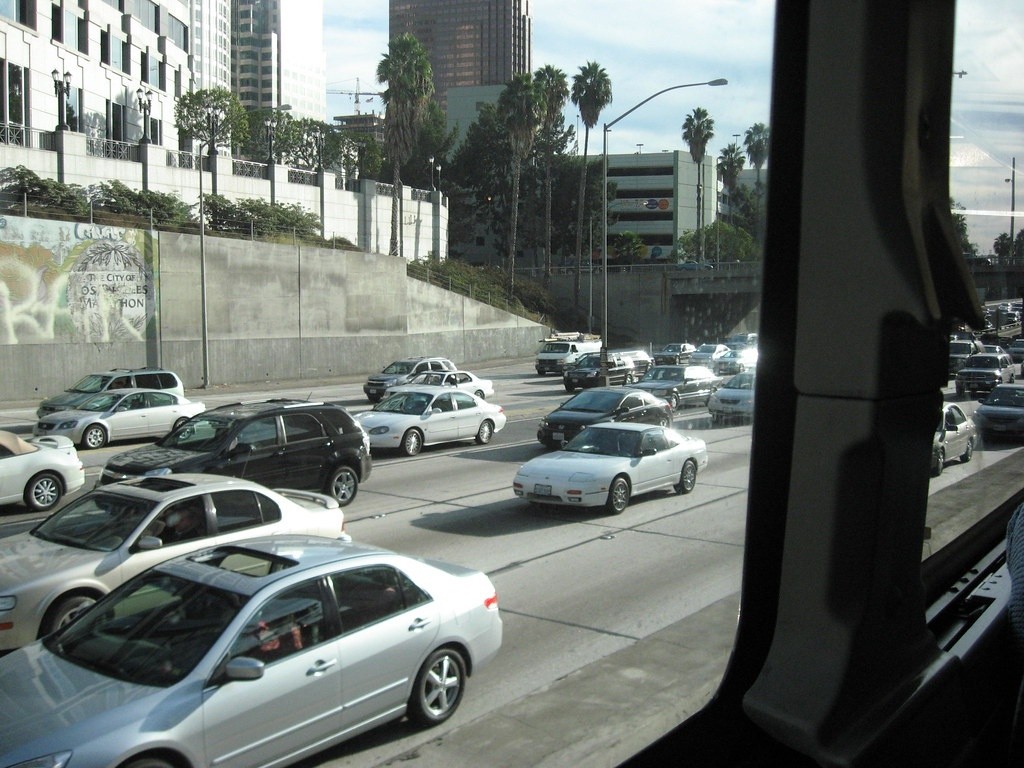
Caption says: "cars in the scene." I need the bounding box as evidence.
[0,431,87,510]
[352,387,506,456]
[1,473,347,655]
[363,356,457,401]
[0,535,503,768]
[562,303,1023,474]
[537,387,674,447]
[383,370,495,399]
[32,386,206,449]
[513,422,709,515]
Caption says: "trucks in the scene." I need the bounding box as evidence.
[535,332,602,374]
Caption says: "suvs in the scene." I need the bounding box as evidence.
[37,367,185,419]
[96,398,372,506]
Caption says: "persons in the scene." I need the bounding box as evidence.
[255,605,301,663]
[163,512,195,545]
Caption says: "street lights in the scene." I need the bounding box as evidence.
[636,143,643,153]
[51,69,73,183]
[313,127,327,240]
[733,135,740,153]
[263,117,279,207]
[135,87,153,189]
[204,102,222,229]
[600,77,727,354]
[1003,157,1016,239]
[355,138,442,192]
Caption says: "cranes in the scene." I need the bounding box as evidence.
[326,76,385,116]
